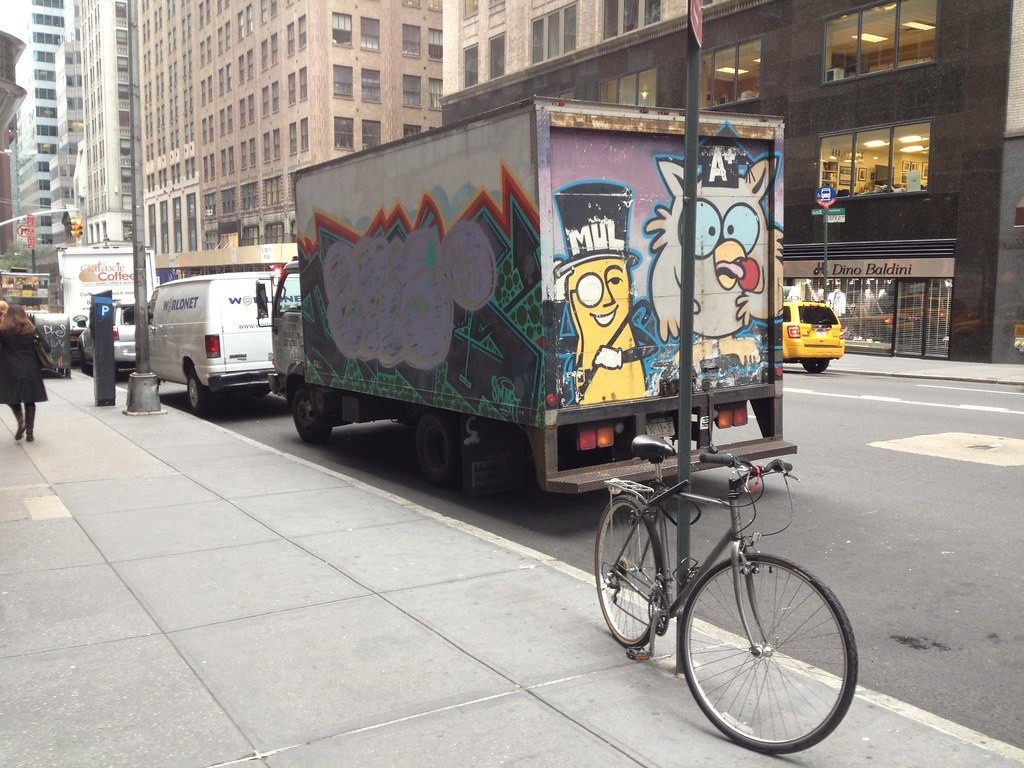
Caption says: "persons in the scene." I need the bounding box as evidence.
[0,300,48,441]
[809,287,890,344]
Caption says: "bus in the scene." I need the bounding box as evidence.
[0,271,52,311]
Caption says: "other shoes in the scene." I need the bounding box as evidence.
[26,432,34,442]
[15,426,25,440]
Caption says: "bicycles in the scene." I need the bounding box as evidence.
[592,436,860,754]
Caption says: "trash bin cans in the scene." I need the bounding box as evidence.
[30,311,45,321]
[25,312,32,316]
[32,312,73,378]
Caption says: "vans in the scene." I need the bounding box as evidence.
[145,269,302,417]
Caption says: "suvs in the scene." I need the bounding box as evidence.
[839,294,950,341]
[779,300,845,374]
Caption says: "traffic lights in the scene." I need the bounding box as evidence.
[76,219,84,238]
[69,218,78,238]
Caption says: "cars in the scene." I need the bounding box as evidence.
[76,295,149,381]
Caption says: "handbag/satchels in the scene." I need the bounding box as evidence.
[32,331,53,368]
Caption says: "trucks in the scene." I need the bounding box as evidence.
[49,242,155,358]
[252,92,797,503]
